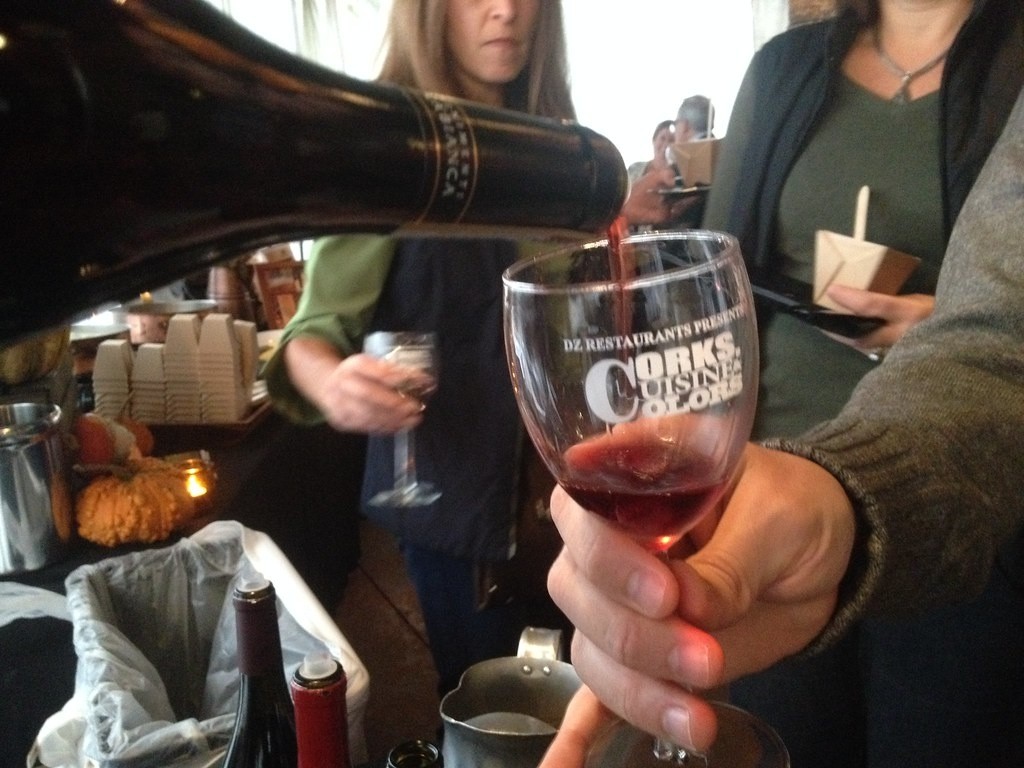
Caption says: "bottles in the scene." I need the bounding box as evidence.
[0,0,629,355]
[290,649,354,768]
[222,576,298,768]
[386,739,443,768]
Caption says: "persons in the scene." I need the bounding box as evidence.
[260,0,1024,768]
[541,93,1024,768]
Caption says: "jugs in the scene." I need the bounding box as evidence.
[439,626,582,768]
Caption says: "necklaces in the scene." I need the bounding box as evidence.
[868,18,953,103]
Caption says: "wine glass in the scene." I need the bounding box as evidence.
[502,229,792,768]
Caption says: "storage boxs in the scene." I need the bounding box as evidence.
[668,136,724,188]
[92,313,262,425]
[813,230,921,316]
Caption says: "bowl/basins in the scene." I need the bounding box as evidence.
[108,300,219,344]
[69,324,132,374]
[0,401,78,576]
